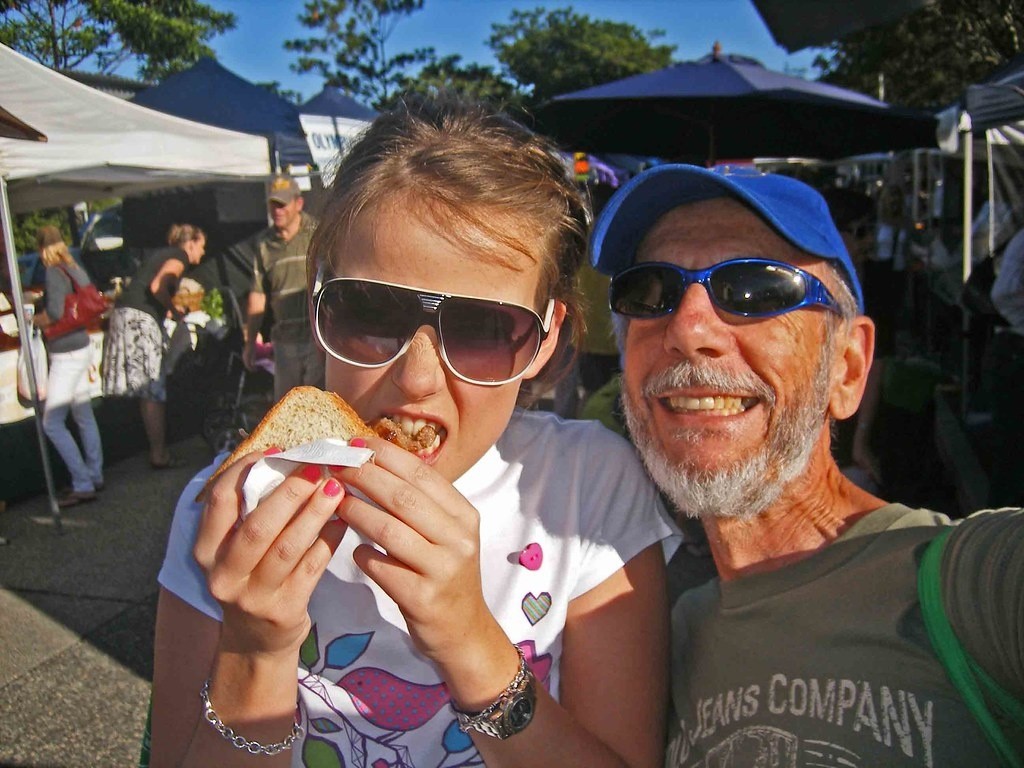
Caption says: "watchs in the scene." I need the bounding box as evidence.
[447,644,536,741]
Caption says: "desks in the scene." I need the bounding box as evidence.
[0,306,230,506]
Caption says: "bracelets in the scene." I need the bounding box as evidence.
[199,678,305,757]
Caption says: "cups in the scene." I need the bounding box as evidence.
[22,304,35,317]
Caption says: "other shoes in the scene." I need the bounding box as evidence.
[58,490,96,508]
[97,482,105,492]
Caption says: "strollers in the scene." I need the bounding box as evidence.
[198,335,271,457]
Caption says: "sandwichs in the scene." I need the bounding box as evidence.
[193,385,440,502]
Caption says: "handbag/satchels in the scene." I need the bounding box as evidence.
[41,264,108,340]
[18,318,48,400]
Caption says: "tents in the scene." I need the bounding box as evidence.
[0,41,386,539]
[843,71,1024,415]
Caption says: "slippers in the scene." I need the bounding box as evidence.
[154,454,187,467]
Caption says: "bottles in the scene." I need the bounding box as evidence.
[933,179,942,214]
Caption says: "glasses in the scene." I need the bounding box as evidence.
[311,260,555,386]
[609,260,846,319]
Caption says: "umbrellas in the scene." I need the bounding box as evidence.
[514,40,943,173]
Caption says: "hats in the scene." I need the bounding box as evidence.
[589,164,865,315]
[265,174,300,205]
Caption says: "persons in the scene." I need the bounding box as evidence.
[103,225,206,471]
[244,174,322,405]
[831,189,891,501]
[590,164,1023,767]
[24,225,105,505]
[149,93,674,766]
